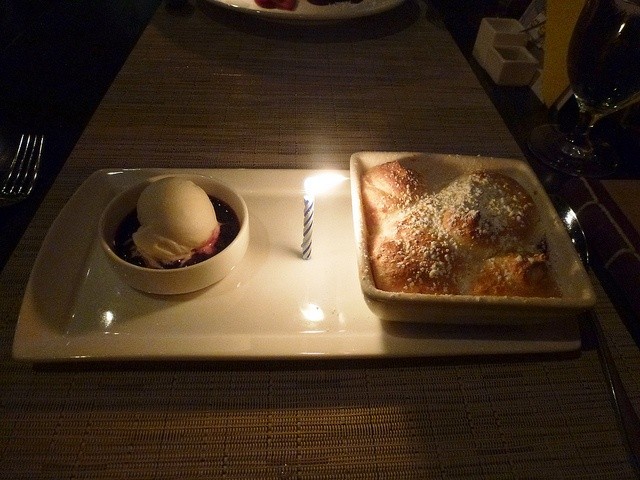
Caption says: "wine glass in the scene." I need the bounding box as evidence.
[527,1,640,176]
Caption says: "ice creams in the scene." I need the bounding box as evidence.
[112,175,239,268]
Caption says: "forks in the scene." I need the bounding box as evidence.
[1,133,46,208]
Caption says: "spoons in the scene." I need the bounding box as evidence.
[545,193,639,477]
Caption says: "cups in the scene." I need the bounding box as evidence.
[472,17,528,67]
[487,45,539,87]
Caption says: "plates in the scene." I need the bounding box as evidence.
[11,169,581,363]
[350,150,598,325]
[211,0,404,25]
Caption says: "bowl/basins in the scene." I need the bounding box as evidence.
[98,172,250,297]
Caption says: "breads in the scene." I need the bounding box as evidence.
[361,160,564,298]
